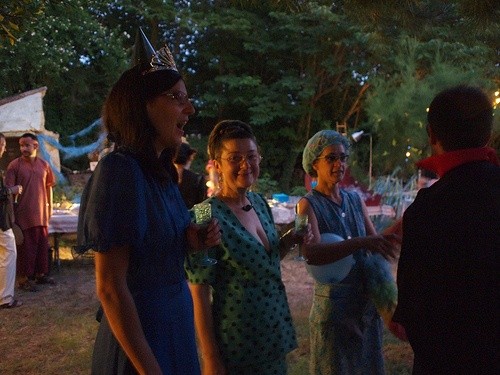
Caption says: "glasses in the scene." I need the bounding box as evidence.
[316,151,349,162]
[216,154,262,162]
[160,90,192,105]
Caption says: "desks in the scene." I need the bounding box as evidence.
[266,196,302,258]
[368,204,396,231]
[47,203,80,273]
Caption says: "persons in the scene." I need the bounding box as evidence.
[185,121,314,375]
[175,142,208,208]
[297,129,401,375]
[77,63,222,375]
[0,132,23,311]
[390,86,500,375]
[5,133,57,290]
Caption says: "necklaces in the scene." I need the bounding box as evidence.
[233,202,254,211]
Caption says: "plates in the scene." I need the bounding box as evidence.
[51,203,80,216]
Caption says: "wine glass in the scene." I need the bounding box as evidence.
[13,184,22,204]
[193,203,217,266]
[293,215,310,261]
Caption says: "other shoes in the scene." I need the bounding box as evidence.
[0,300,24,308]
[36,275,56,285]
[20,280,39,293]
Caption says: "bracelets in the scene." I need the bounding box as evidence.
[281,225,295,253]
[10,186,16,194]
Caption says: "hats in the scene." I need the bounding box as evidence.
[302,130,351,175]
